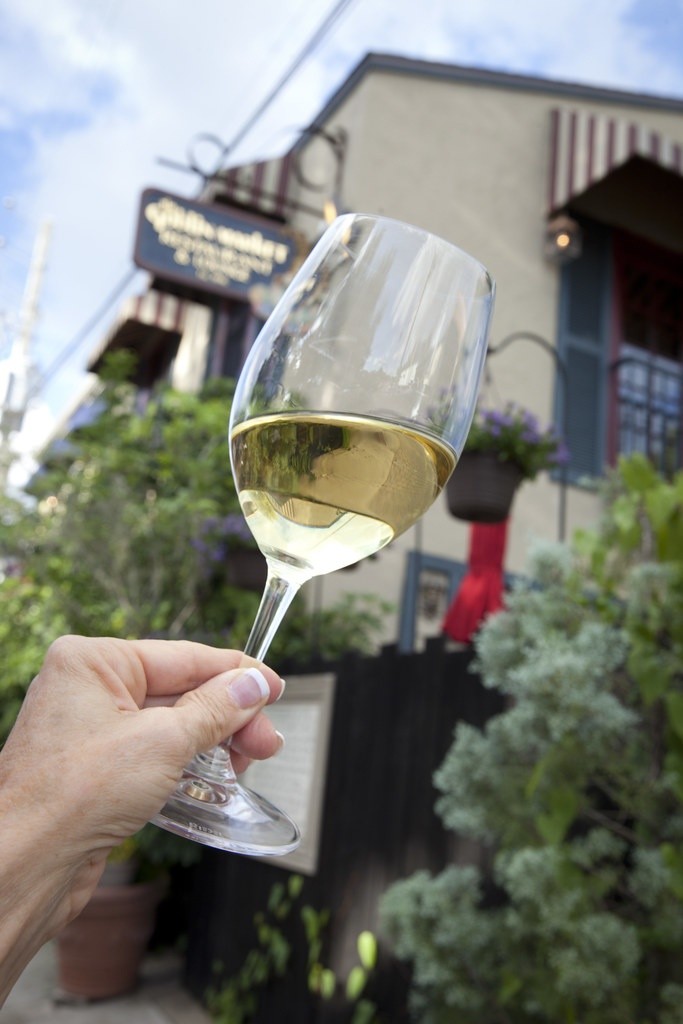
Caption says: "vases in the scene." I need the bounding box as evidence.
[446,459,523,525]
[49,872,171,998]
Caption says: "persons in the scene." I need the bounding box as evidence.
[0,636,286,1024]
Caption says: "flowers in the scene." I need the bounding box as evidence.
[426,386,572,478]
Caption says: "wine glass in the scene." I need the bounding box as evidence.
[147,213,494,857]
